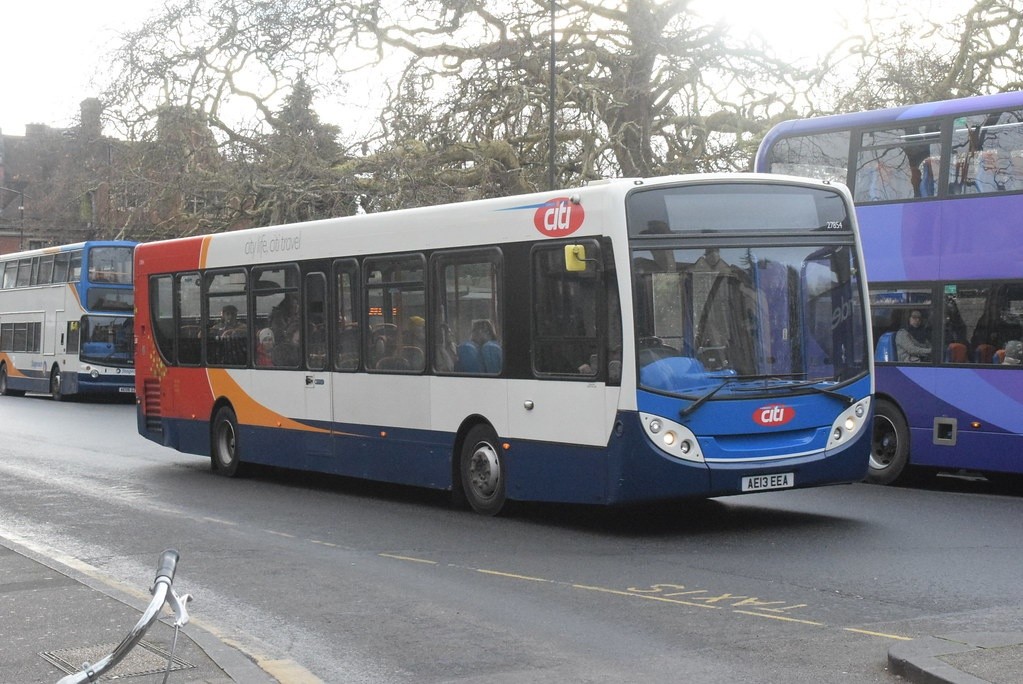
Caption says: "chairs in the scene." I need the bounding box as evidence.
[177,324,502,374]
[918,149,1023,198]
[873,330,1006,365]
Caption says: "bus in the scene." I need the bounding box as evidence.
[0,240,141,403]
[133,171,876,520]
[754,89,1021,489]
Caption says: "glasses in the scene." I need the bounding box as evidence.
[910,315,923,320]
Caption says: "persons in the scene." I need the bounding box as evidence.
[898,298,1023,366]
[216,298,498,369]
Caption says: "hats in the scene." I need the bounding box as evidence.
[259,328,275,344]
[409,316,425,327]
